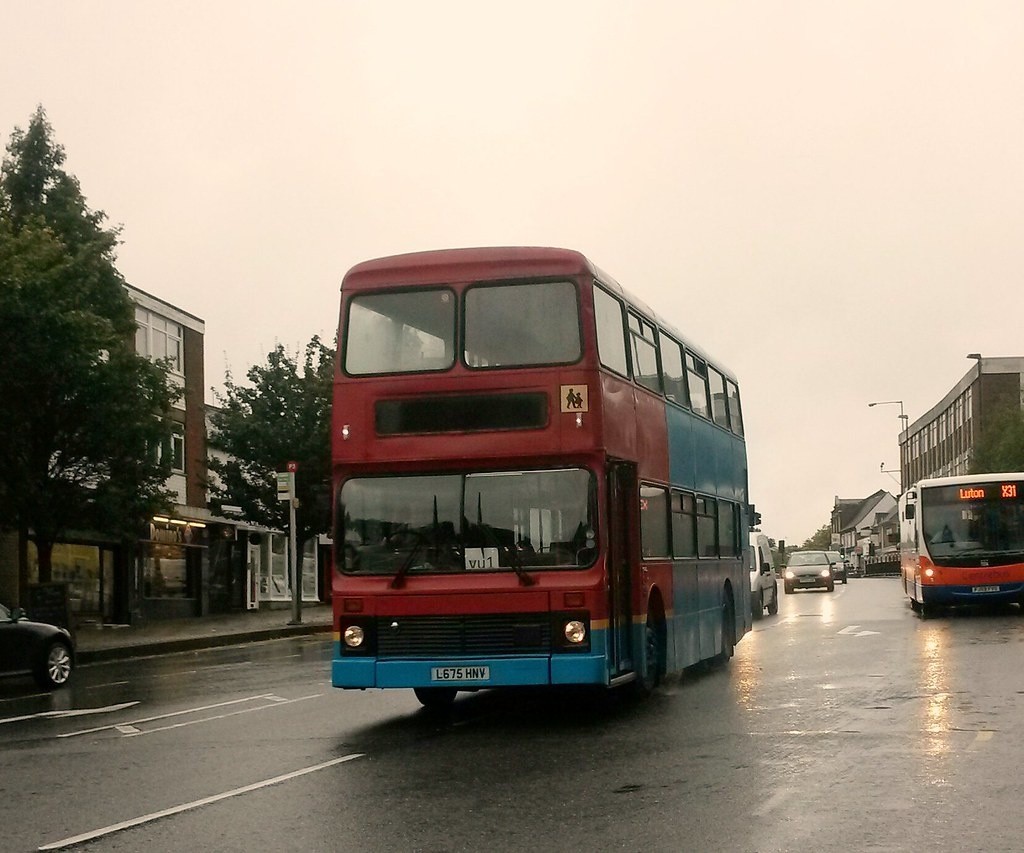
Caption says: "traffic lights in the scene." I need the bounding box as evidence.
[841,548,845,559]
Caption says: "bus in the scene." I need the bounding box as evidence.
[327,244,751,713]
[896,470,1023,618]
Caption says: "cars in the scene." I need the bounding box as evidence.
[0,604,78,689]
[780,551,836,594]
[824,550,850,584]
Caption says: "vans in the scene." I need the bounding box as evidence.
[748,532,778,619]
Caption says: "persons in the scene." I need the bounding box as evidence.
[930,520,961,544]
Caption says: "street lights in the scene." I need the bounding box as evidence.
[868,399,904,430]
[966,353,985,437]
[897,415,910,490]
